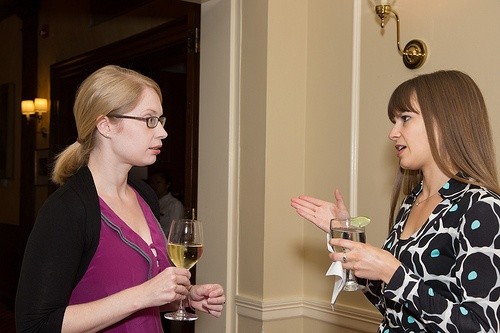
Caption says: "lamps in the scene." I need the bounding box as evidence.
[370,0,427,70]
[21,97,47,134]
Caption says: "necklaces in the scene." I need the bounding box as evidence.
[416,193,438,206]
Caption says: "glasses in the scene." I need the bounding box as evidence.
[106,114,166,129]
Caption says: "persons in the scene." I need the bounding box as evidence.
[149,169,184,240]
[291,70,500,333]
[12,65,225,333]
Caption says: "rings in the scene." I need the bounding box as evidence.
[343,253,346,263]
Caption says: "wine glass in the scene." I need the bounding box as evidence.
[330,218,368,292]
[164,219,204,321]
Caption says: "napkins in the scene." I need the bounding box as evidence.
[326,233,346,305]
